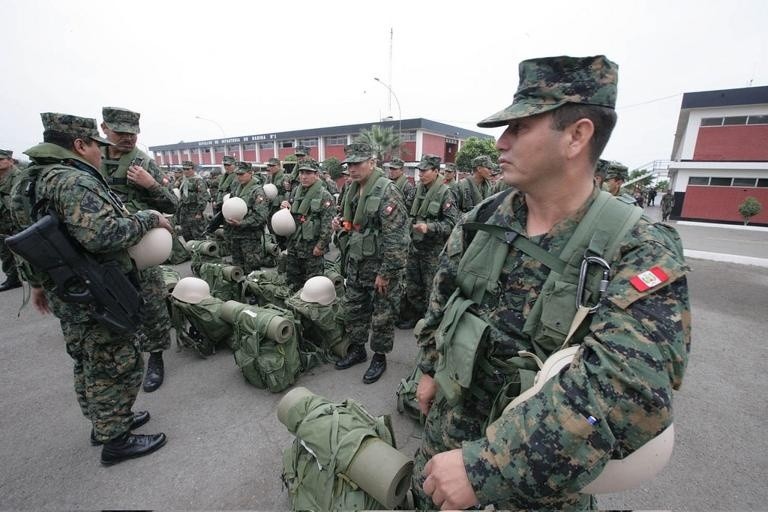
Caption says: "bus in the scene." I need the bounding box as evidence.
[158,161,448,186]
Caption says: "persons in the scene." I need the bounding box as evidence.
[0,106,181,467]
[365,56,692,512]
[143,144,411,386]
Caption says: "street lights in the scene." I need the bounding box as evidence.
[196,115,225,138]
[377,110,393,127]
[374,73,404,163]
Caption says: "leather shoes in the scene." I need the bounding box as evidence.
[363,356,387,384]
[89,410,151,446]
[334,348,368,370]
[142,356,164,392]
[100,431,167,466]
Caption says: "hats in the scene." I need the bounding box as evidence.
[182,160,195,170]
[444,162,457,172]
[476,53,619,128]
[470,155,499,170]
[40,112,116,147]
[0,148,14,159]
[414,153,442,171]
[598,160,630,180]
[102,107,141,135]
[221,143,405,172]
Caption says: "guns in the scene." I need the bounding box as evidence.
[4,215,140,334]
[201,211,224,237]
